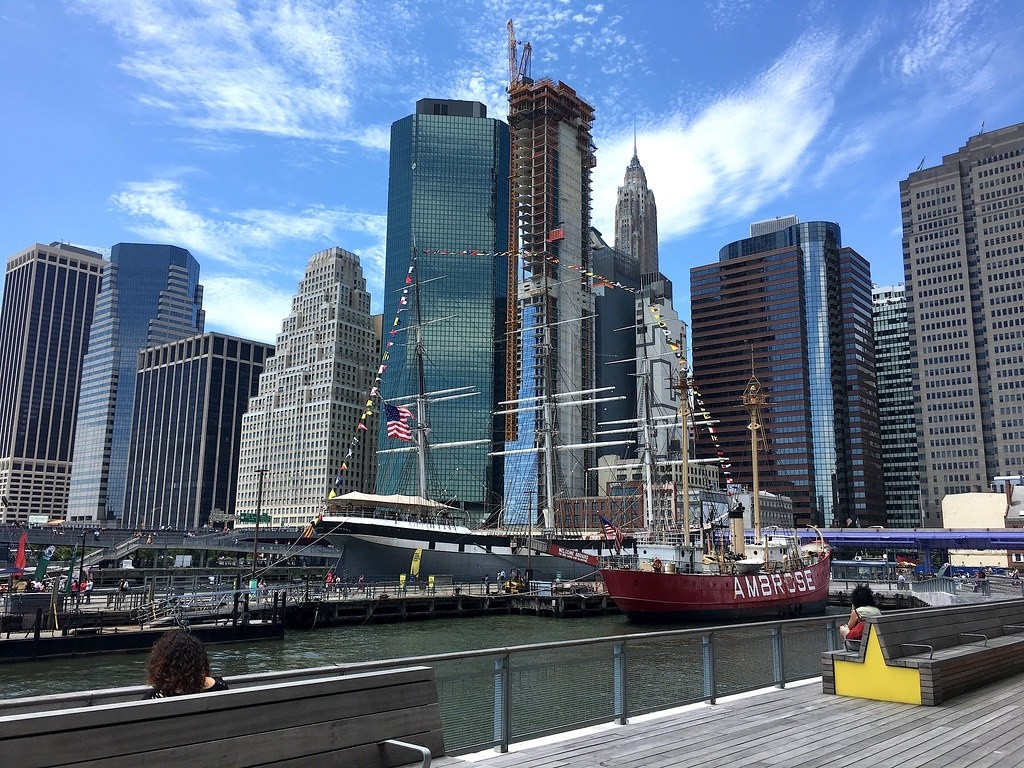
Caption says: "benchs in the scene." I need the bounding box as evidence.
[820,599,1024,706]
[0,667,476,768]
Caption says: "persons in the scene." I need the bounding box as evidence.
[12,576,128,604]
[328,570,366,595]
[896,566,1019,594]
[485,569,506,587]
[142,631,228,700]
[258,578,268,589]
[839,585,883,652]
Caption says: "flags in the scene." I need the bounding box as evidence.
[304,246,739,557]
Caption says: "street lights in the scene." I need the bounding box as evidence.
[918,480,925,528]
[250,469,271,579]
[75,530,87,615]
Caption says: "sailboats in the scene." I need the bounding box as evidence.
[596,344,832,624]
[308,224,790,592]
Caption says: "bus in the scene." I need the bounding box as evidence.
[830,560,916,582]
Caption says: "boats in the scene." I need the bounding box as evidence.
[734,558,765,574]
[1,576,289,660]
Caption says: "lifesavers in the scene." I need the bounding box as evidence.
[818,553,823,559]
[652,558,662,570]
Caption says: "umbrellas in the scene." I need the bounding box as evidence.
[0,567,30,579]
